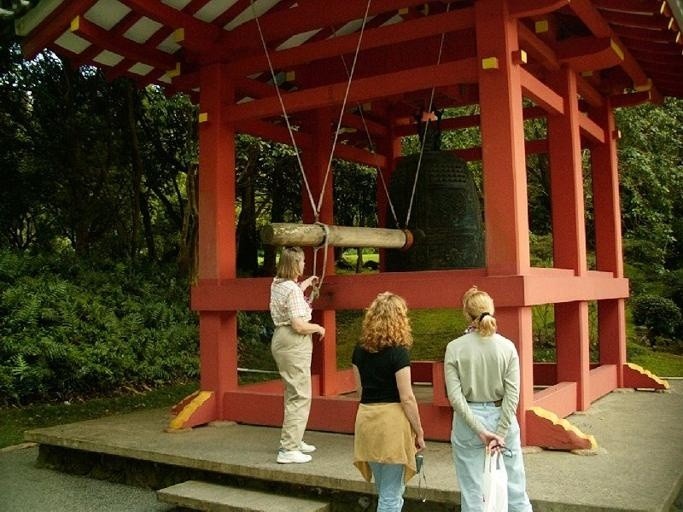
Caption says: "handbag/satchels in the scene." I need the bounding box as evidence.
[482,470,508,512]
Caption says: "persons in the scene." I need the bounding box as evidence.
[352,291,426,512]
[444,285,533,512]
[269,246,325,464]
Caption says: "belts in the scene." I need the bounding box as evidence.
[467,400,502,407]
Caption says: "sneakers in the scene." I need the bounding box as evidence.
[277,440,316,463]
